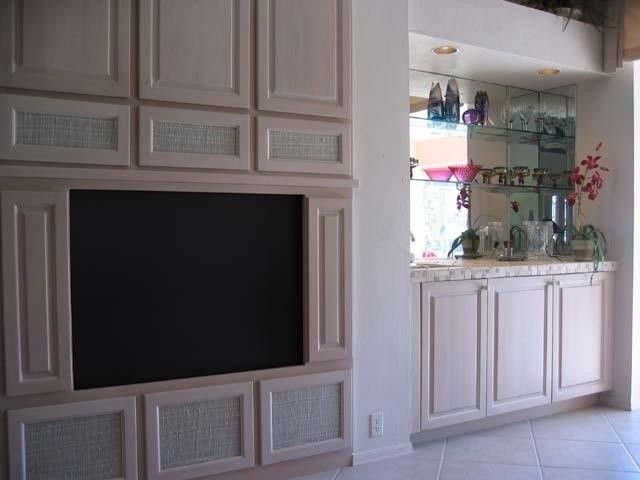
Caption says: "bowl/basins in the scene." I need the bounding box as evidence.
[448,165,483,183]
[422,168,455,181]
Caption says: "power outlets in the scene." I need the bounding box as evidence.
[372,413,383,436]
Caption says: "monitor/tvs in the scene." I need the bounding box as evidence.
[68,189,306,392]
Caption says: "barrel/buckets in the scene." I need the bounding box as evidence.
[524,220,555,258]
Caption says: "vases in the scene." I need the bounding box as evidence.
[444,77,489,126]
[462,235,480,256]
[513,225,528,258]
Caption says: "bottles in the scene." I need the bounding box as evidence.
[462,108,480,124]
[522,218,555,258]
[487,222,504,258]
[475,90,489,126]
[445,76,460,123]
[478,224,487,255]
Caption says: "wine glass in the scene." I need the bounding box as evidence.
[501,102,561,135]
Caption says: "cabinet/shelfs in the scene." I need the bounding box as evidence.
[409,115,575,193]
[486,271,616,430]
[410,278,488,445]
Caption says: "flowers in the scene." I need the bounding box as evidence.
[447,188,485,260]
[555,142,610,286]
[510,201,528,251]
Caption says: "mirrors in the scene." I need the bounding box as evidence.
[410,68,576,268]
